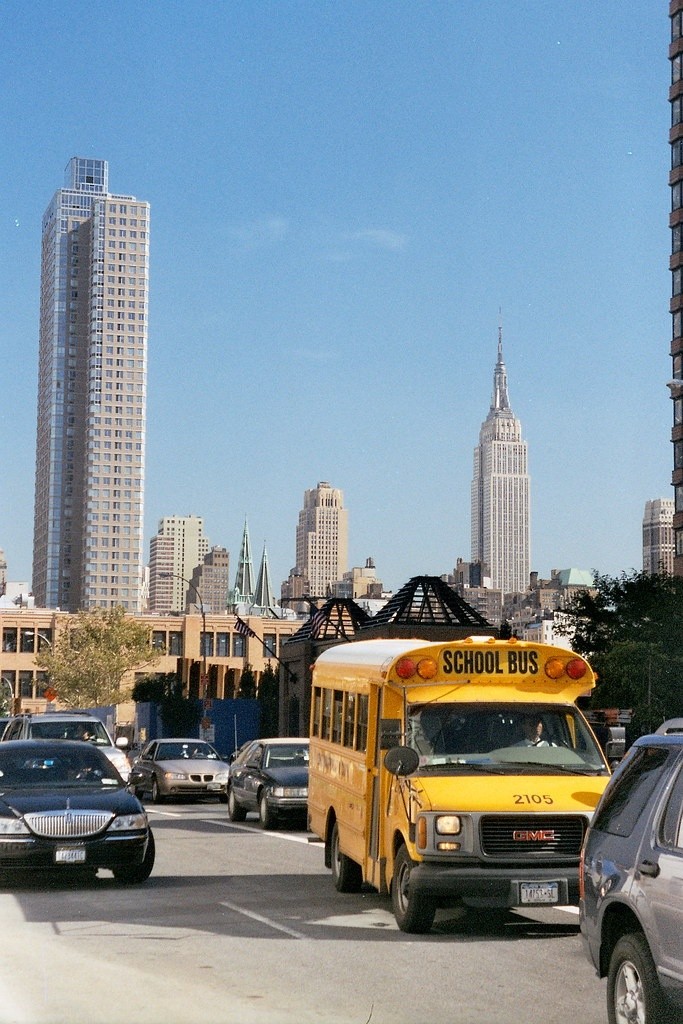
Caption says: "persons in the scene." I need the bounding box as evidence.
[511,713,556,749]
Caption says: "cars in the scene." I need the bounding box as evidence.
[133,738,230,804]
[0,738,156,886]
[229,740,253,765]
[224,737,310,830]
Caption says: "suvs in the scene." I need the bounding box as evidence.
[579,718,683,1024]
[0,711,132,788]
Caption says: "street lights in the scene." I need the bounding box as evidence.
[157,572,207,738]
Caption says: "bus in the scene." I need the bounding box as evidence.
[309,636,613,933]
